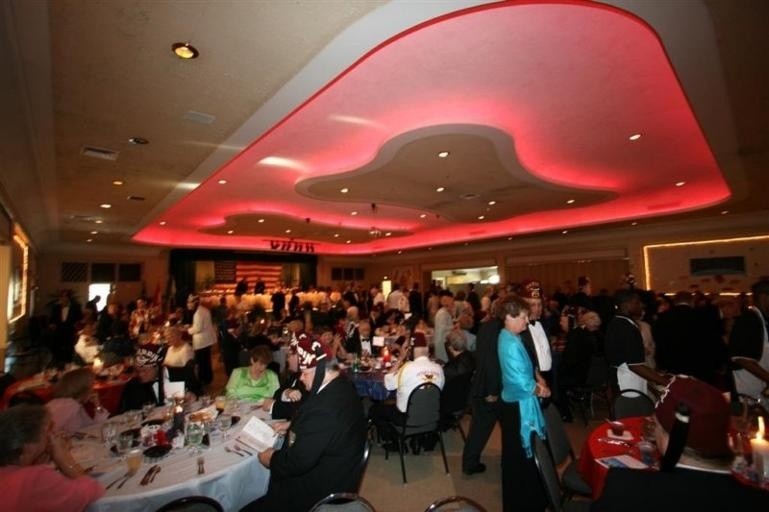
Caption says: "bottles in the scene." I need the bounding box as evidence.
[350,353,360,373]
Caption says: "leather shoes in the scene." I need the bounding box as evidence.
[462,461,486,476]
[382,443,408,454]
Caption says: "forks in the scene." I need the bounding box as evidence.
[224,443,252,457]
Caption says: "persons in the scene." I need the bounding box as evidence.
[308,276,769,512]
[0,276,368,512]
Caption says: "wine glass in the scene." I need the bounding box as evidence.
[727,412,755,478]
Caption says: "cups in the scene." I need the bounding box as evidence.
[34,361,126,387]
[100,393,232,473]
[638,416,660,467]
[611,421,624,436]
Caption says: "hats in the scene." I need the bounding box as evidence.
[518,280,544,299]
[185,293,199,306]
[287,329,312,356]
[297,338,337,373]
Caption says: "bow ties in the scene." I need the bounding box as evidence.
[361,338,369,342]
[528,320,536,327]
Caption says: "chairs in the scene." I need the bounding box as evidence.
[530,429,592,512]
[309,492,375,511]
[542,399,594,501]
[437,370,477,443]
[607,388,655,422]
[382,382,452,483]
[421,495,487,512]
[156,495,224,511]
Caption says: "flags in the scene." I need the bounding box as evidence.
[214,261,282,293]
